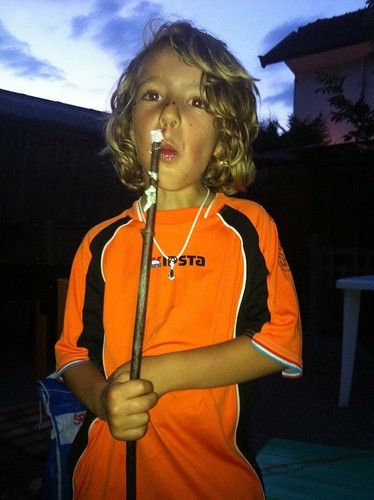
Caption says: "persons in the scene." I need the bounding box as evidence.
[55,21,303,500]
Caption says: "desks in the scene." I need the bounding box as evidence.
[335,276,374,408]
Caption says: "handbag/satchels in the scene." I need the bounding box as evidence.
[36,360,104,500]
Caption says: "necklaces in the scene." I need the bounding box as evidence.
[138,188,211,279]
